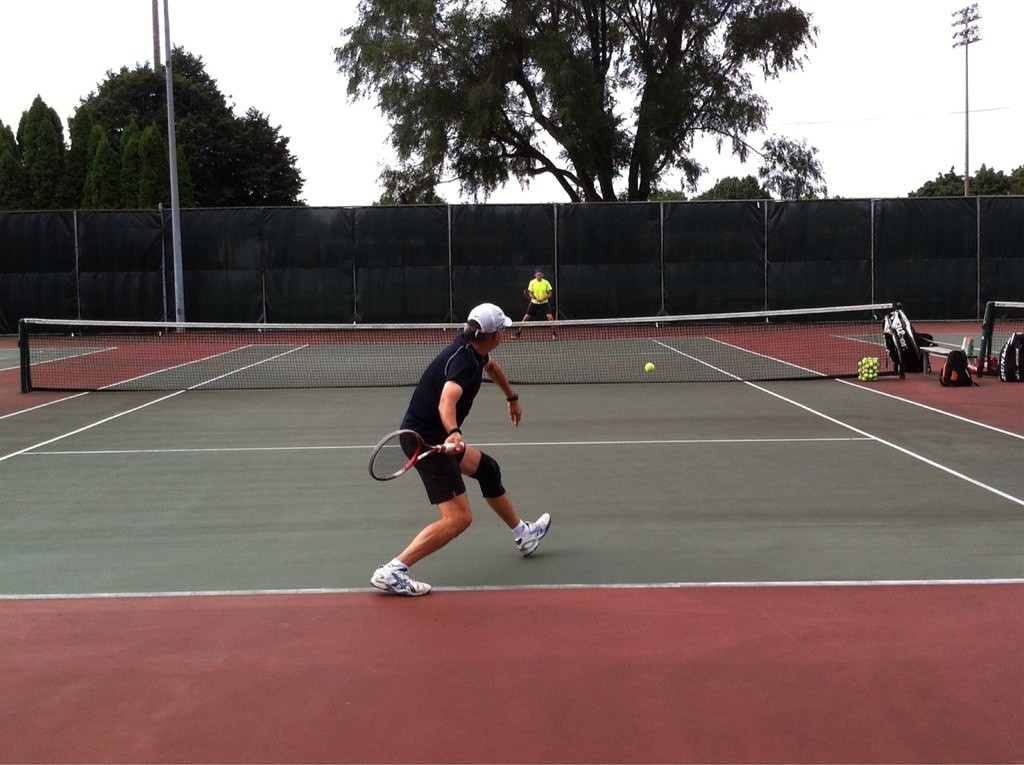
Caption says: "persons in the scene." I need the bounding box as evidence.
[369,301,552,597]
[510,268,558,340]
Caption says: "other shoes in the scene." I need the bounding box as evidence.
[551,334,559,342]
[511,333,521,339]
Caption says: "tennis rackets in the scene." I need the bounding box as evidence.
[368,429,465,482]
[524,288,538,301]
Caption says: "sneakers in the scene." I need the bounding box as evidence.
[370,564,432,596]
[514,512,552,556]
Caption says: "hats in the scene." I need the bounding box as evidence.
[467,303,513,337]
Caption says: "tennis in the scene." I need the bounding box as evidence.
[645,362,656,373]
[857,356,881,382]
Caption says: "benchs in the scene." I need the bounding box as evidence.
[920,346,953,380]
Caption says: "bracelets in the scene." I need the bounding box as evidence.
[447,428,462,436]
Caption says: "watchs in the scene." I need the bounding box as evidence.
[506,393,519,402]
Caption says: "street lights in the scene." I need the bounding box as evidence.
[948,1,984,194]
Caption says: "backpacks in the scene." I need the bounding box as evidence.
[998,332,1024,383]
[881,310,934,373]
[939,351,974,387]
